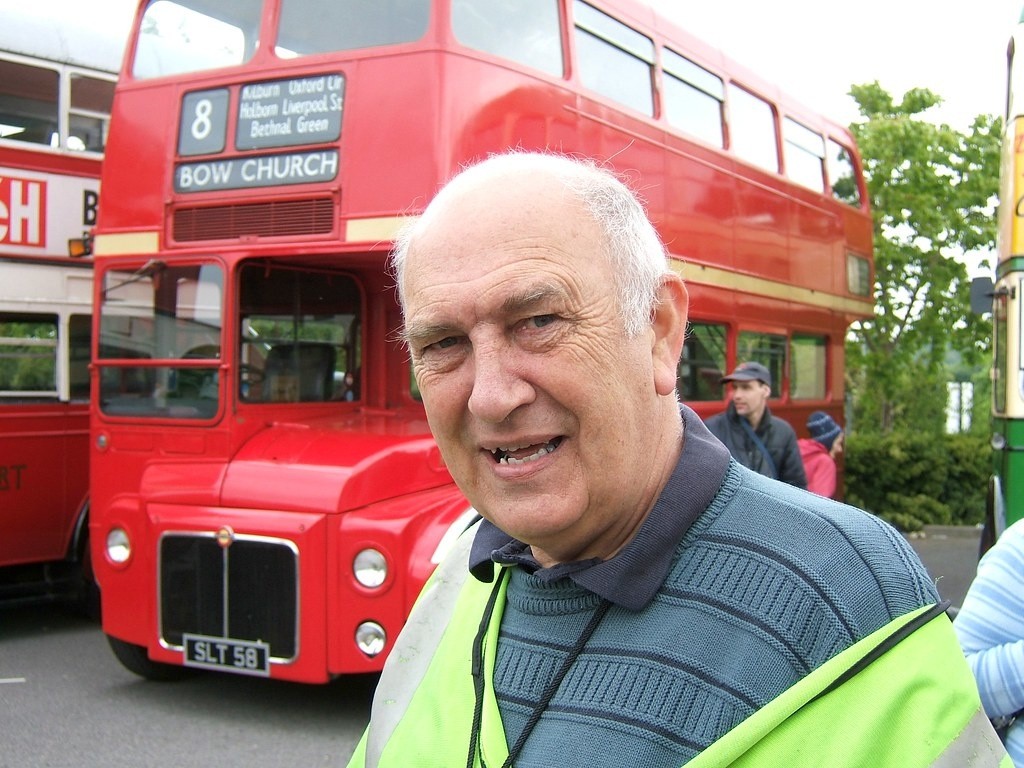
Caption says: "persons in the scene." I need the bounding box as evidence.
[952,518,1024,767]
[702,361,843,498]
[345,153,1015,768]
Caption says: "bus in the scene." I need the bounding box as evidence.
[0,18,221,616]
[971,15,1024,560]
[90,0,874,685]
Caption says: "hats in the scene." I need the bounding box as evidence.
[719,362,771,388]
[806,412,841,452]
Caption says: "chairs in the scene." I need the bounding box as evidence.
[260,343,338,402]
